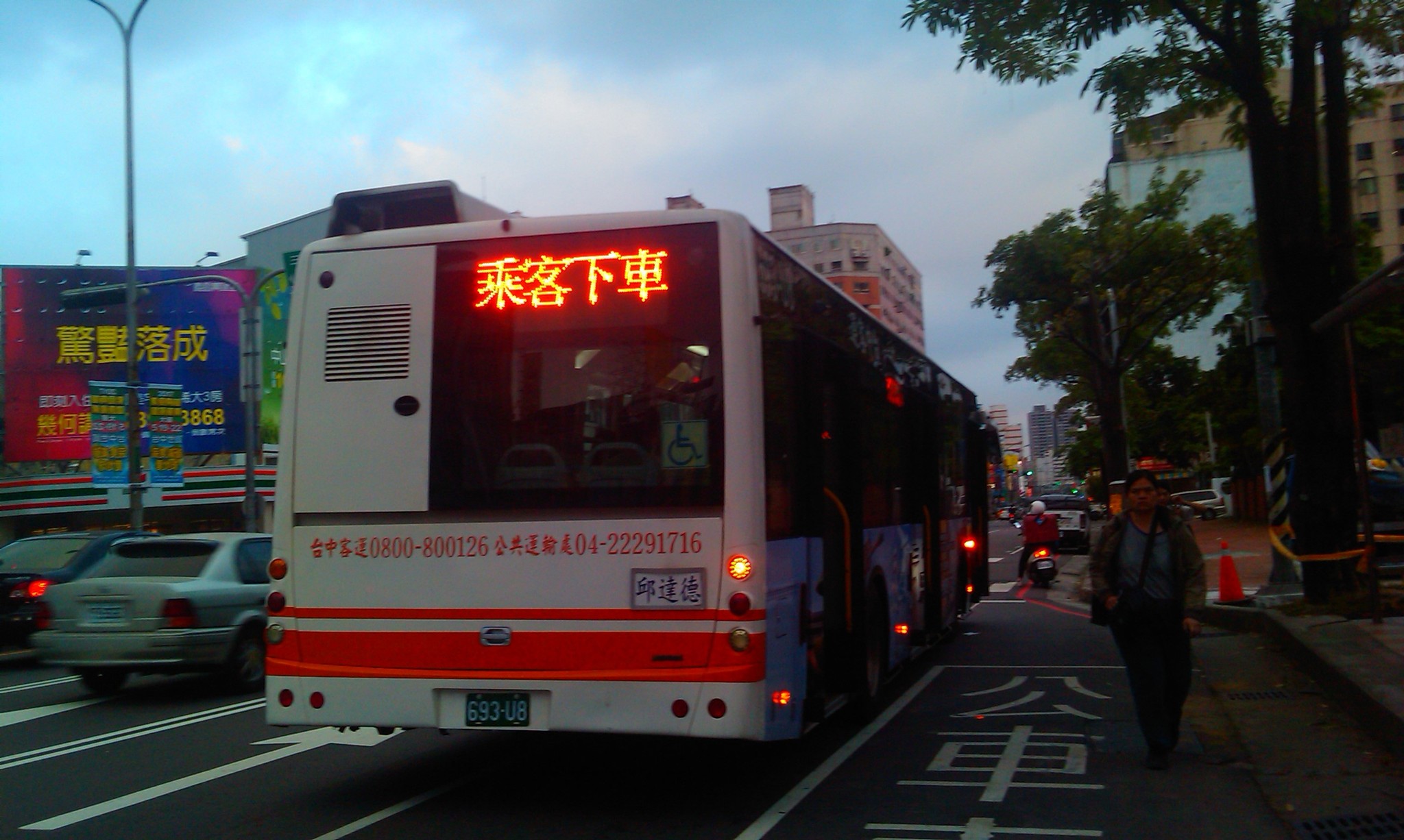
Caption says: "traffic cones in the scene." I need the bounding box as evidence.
[1213,539,1255,604]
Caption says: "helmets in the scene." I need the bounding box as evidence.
[1030,501,1046,514]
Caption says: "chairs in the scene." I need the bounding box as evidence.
[576,442,659,489]
[494,442,570,488]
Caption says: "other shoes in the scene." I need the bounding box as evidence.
[1148,753,1168,769]
[1165,744,1182,764]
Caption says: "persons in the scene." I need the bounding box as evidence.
[1155,482,1206,519]
[1009,505,1016,514]
[1016,501,1060,587]
[1088,469,1208,774]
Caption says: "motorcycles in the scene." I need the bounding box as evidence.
[1013,521,1061,588]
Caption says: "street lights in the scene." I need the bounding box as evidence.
[60,265,291,534]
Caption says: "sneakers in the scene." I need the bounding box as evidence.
[1016,577,1024,587]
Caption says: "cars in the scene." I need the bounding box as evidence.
[24,528,274,700]
[992,492,1093,555]
[1169,488,1228,521]
[1,530,168,656]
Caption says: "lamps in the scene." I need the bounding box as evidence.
[75,250,90,266]
[195,252,218,266]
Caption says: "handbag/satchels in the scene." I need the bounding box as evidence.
[1092,594,1123,627]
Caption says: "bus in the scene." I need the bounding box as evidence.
[258,194,1006,754]
